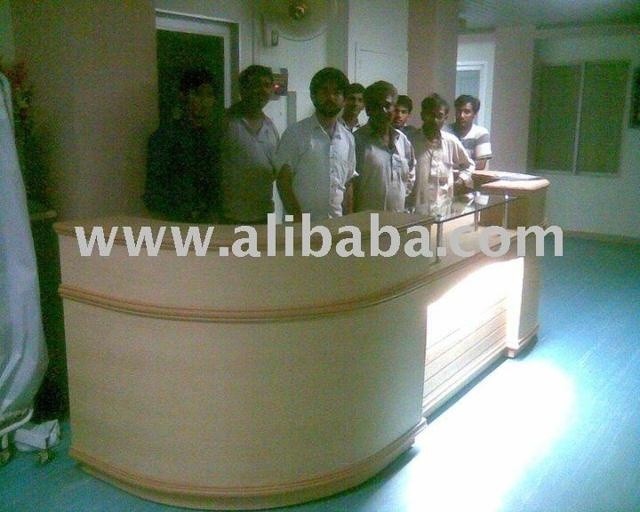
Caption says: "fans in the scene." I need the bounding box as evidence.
[244,0,340,47]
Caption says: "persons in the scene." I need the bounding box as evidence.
[351,80,417,213]
[216,64,280,226]
[390,95,418,208]
[275,66,359,224]
[442,94,492,196]
[336,83,366,132]
[142,68,221,224]
[408,92,476,205]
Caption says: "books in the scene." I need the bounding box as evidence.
[495,171,542,182]
